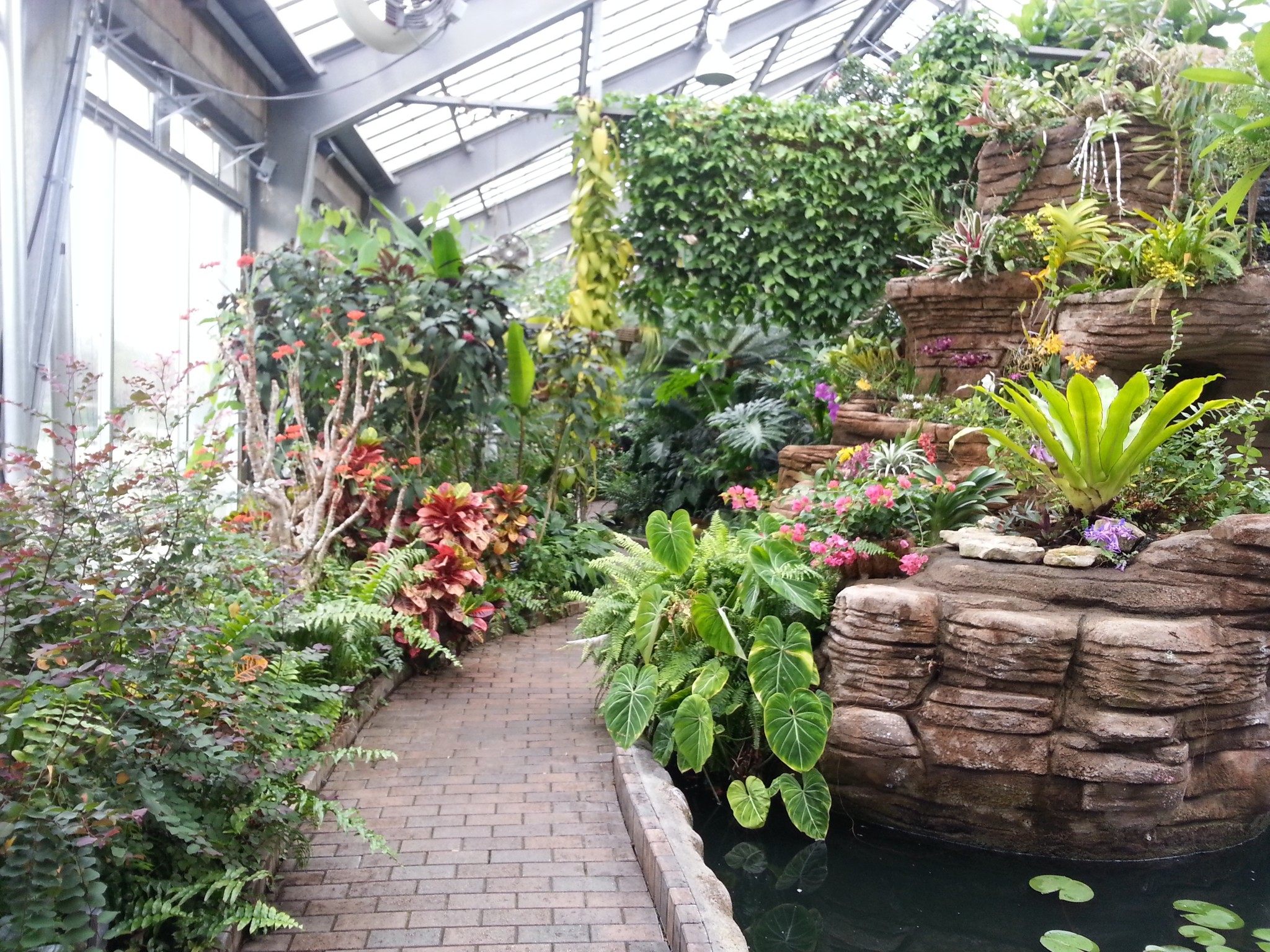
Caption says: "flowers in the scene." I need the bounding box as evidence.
[741,314,1127,584]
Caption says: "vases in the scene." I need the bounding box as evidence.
[847,528,916,574]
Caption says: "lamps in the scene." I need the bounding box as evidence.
[694,13,737,83]
